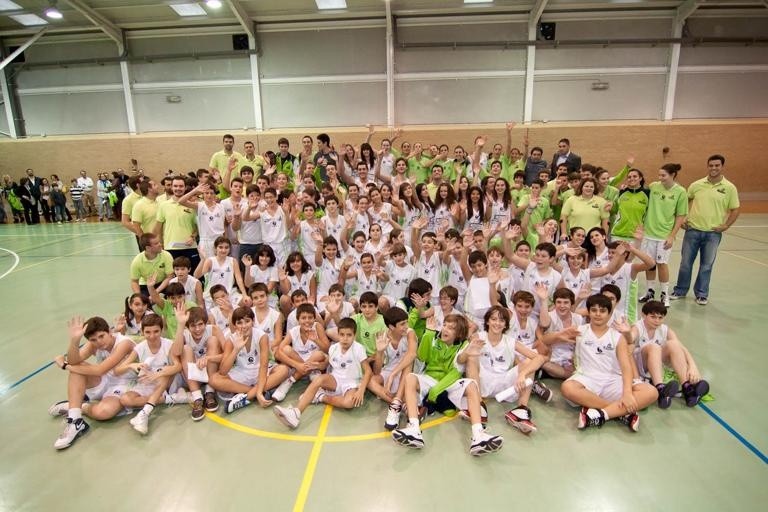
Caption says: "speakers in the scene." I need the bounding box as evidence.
[232,35,249,50]
[540,23,555,40]
[9,46,25,63]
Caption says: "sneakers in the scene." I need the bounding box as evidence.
[682,382,709,406]
[505,405,537,437]
[459,402,488,423]
[639,289,707,307]
[302,387,324,403]
[53,416,90,450]
[167,383,300,430]
[619,413,640,430]
[657,380,680,407]
[384,399,428,448]
[531,380,553,401]
[130,411,149,434]
[49,402,70,417]
[578,407,605,429]
[469,430,503,457]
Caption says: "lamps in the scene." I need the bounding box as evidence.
[45,1,64,22]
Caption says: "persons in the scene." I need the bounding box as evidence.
[2,160,151,226]
[48,122,740,456]
